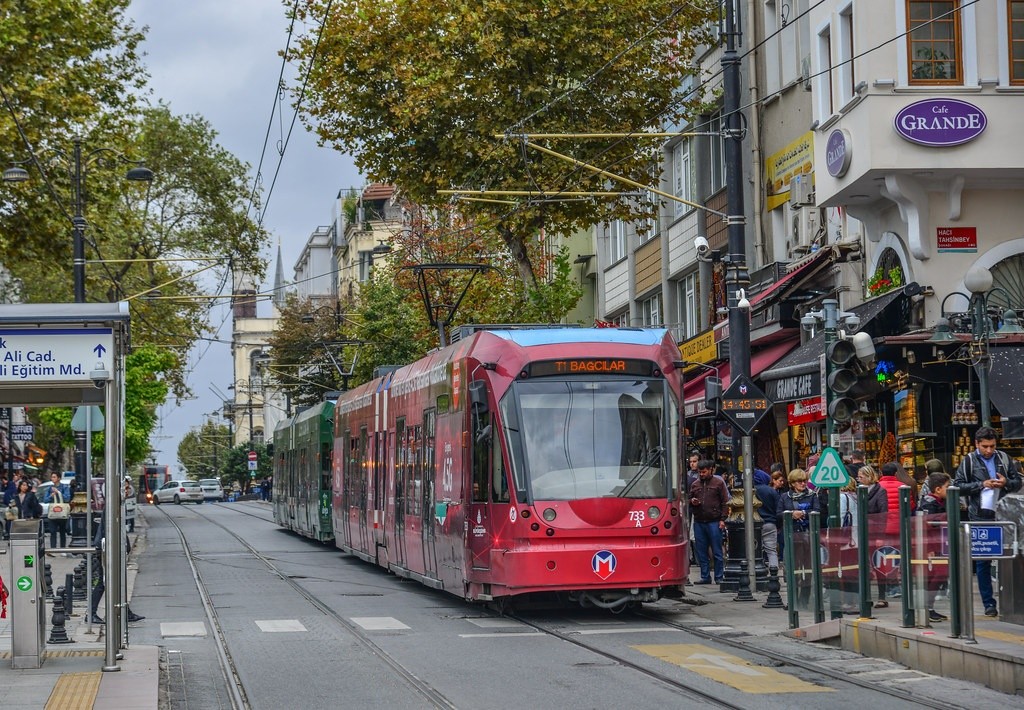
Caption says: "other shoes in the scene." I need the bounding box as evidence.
[46,553,56,557]
[985,606,997,616]
[61,552,67,556]
[716,578,723,583]
[784,603,807,610]
[694,578,711,584]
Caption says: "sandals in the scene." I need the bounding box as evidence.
[874,601,888,608]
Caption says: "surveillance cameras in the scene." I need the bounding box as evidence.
[800,317,816,331]
[845,317,860,331]
[738,299,750,313]
[694,236,710,255]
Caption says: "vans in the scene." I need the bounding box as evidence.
[35,476,76,535]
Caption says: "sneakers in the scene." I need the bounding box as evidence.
[85,614,105,623]
[128,609,145,620]
[929,610,941,622]
[933,610,947,619]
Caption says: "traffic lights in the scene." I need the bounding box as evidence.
[826,340,858,423]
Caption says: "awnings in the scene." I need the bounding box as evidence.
[970,346,1024,439]
[760,290,902,403]
[683,339,799,418]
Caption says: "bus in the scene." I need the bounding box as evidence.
[273,391,346,544]
[333,324,722,602]
[138,464,168,503]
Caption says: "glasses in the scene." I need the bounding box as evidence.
[794,480,806,483]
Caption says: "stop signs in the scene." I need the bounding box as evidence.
[248,452,257,461]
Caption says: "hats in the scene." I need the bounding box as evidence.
[125,476,132,482]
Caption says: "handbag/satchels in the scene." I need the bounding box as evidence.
[47,491,70,519]
[840,494,852,545]
[37,504,43,516]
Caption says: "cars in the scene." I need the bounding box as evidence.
[152,480,204,505]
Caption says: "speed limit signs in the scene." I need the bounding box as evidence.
[811,448,850,487]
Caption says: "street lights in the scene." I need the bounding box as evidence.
[925,264,1024,432]
[1,122,155,600]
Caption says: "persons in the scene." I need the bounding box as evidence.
[682,452,967,622]
[260,475,273,501]
[83,477,143,624]
[954,427,1022,616]
[0,470,76,557]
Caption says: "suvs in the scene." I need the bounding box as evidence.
[198,479,223,502]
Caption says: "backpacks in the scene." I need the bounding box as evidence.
[263,482,269,488]
